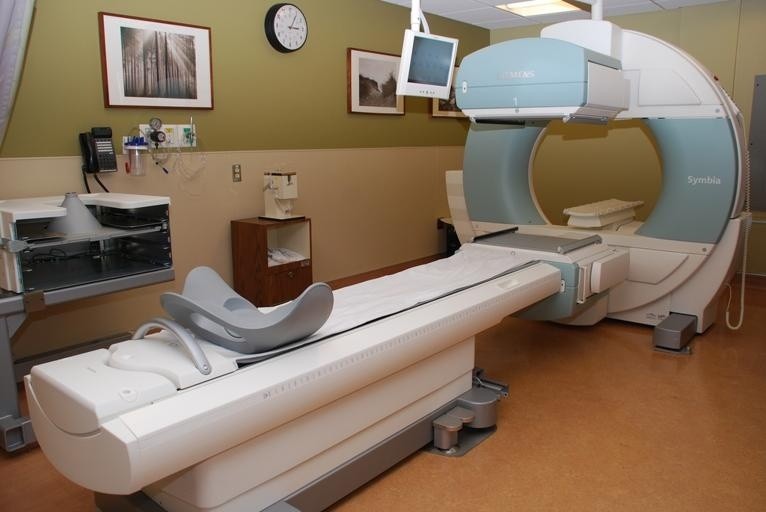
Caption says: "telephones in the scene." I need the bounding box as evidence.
[79,126,117,173]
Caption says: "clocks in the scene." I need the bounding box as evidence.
[264,2,309,53]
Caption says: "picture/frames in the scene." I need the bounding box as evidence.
[97,10,214,110]
[346,48,468,119]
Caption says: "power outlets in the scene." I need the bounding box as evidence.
[138,124,196,150]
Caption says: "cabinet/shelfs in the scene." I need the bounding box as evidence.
[230,214,313,311]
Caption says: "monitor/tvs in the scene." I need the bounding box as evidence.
[394,29,460,101]
